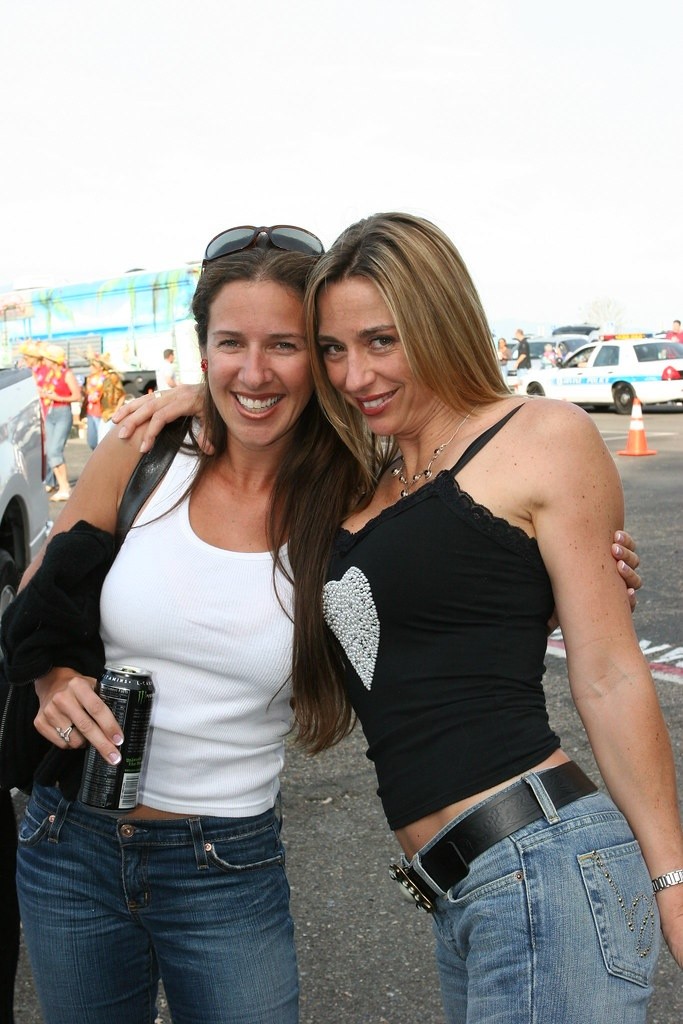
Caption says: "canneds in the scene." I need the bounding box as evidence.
[77,666,157,814]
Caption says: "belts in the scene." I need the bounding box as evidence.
[388,760,601,914]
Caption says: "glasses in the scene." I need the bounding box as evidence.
[200,225,325,275]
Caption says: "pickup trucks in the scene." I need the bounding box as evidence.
[0,368,54,622]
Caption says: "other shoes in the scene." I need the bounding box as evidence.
[45,484,55,494]
[49,489,72,501]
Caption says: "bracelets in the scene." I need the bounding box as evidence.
[653,870,683,892]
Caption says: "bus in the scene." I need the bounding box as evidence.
[0,264,203,401]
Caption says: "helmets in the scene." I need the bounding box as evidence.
[41,343,65,364]
[17,340,43,360]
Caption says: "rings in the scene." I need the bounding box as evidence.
[55,724,75,743]
[154,390,161,398]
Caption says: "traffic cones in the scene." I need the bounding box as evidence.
[614,398,659,458]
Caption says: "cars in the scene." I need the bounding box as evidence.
[550,323,610,338]
[506,333,683,413]
[501,335,593,387]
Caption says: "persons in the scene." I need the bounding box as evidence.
[14,211,683,1024]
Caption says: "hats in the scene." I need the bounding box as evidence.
[84,352,114,370]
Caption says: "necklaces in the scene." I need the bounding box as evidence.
[40,367,62,406]
[87,373,105,409]
[389,405,480,498]
[31,362,40,373]
